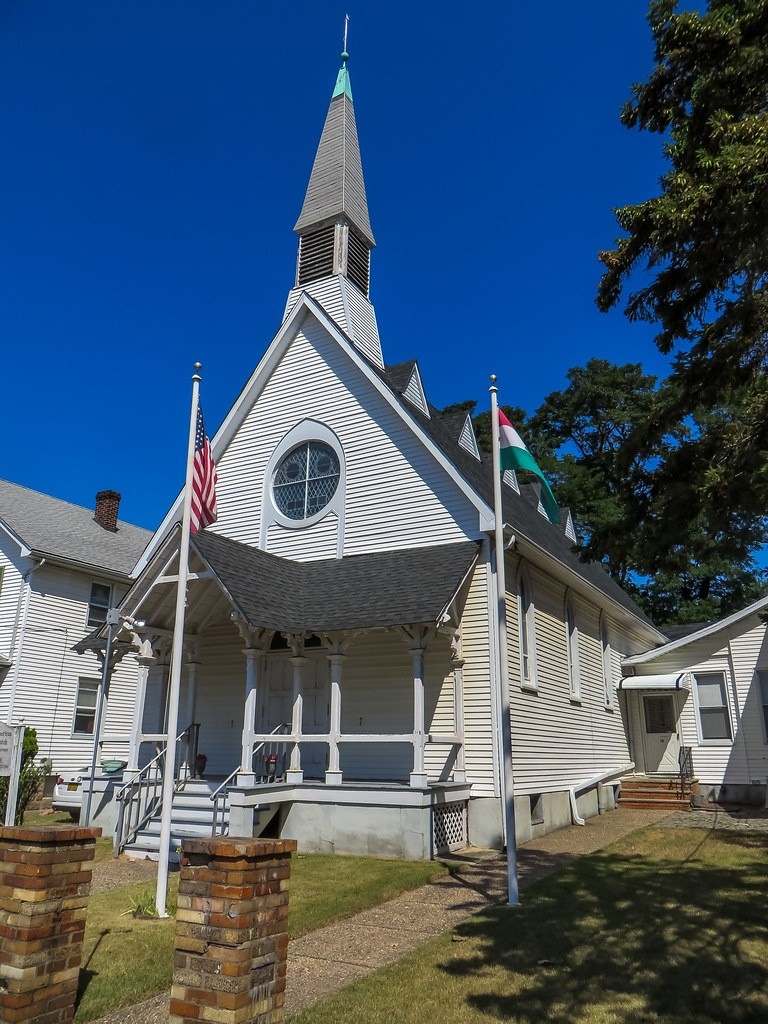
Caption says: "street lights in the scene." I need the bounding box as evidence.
[85,607,121,828]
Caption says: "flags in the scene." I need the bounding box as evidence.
[498,407,564,525]
[189,394,218,535]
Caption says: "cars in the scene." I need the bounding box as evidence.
[50,766,96,820]
